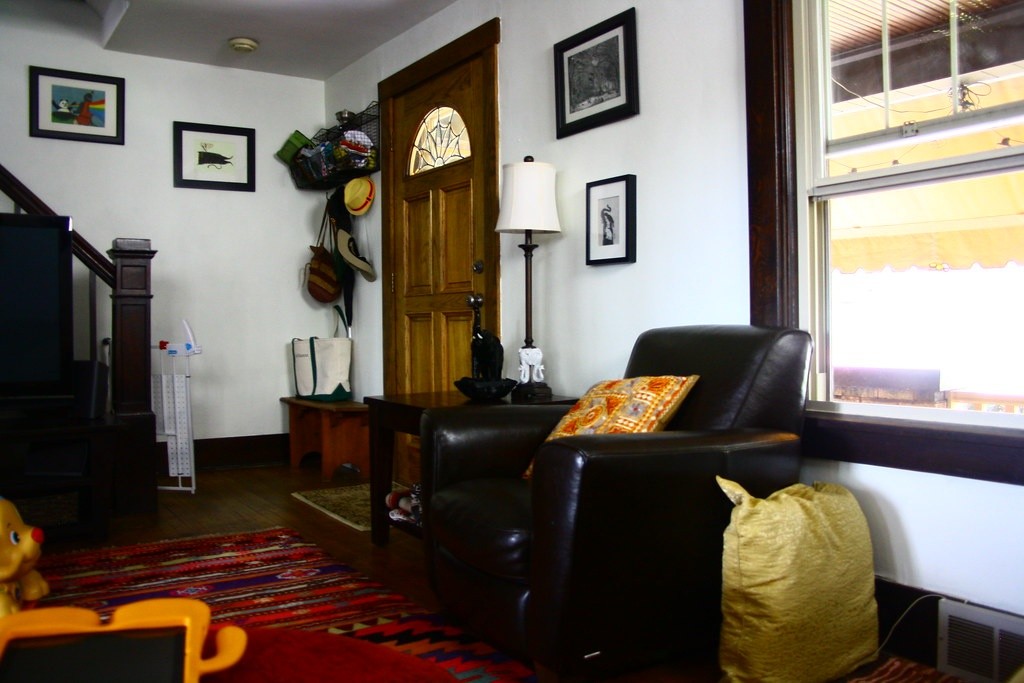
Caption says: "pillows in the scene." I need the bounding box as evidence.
[522,375,701,482]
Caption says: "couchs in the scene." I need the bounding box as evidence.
[418,325,815,674]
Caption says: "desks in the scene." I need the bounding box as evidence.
[1,413,128,540]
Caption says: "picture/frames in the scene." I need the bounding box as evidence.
[29,65,125,145]
[553,6,640,139]
[173,121,256,192]
[585,174,637,266]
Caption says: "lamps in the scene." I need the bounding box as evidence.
[495,156,565,403]
[229,38,258,51]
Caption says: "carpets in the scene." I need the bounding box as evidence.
[291,481,412,532]
[35,525,548,683]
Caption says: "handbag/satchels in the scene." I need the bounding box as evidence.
[291,305,353,402]
[303,200,342,303]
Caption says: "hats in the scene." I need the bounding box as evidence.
[345,176,375,216]
[337,229,376,282]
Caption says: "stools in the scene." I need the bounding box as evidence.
[280,394,370,481]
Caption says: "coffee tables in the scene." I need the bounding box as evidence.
[363,388,580,593]
